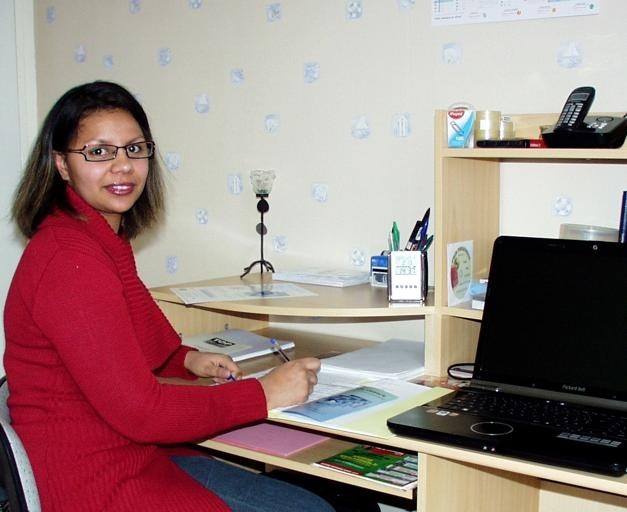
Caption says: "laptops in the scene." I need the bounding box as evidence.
[386,235,627,477]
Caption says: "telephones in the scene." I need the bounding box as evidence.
[541,87,626,148]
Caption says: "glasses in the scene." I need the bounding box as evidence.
[59,140,156,162]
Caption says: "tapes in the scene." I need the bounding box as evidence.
[474,109,514,141]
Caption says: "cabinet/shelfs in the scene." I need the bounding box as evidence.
[148,109,627,378]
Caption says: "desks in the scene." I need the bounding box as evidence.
[148,327,625,512]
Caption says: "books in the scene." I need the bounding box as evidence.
[312,442,418,489]
[272,267,370,288]
[175,327,295,363]
[204,337,462,457]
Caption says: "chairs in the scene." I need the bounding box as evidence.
[0,374,43,510]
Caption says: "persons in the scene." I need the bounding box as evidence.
[4,82,337,512]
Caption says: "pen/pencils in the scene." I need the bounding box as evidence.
[391,208,433,252]
[270,339,289,363]
